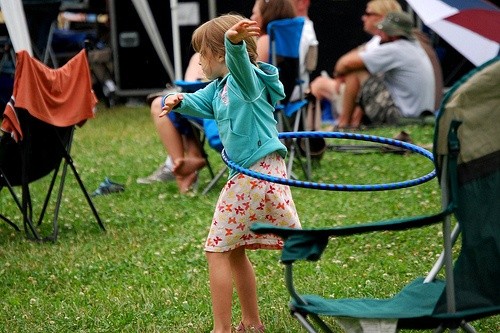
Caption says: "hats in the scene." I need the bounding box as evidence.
[374,12,415,42]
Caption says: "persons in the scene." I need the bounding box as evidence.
[158,14,304,332]
[414,27,444,113]
[335,10,435,129]
[298,0,407,128]
[135,49,208,186]
[150,0,297,196]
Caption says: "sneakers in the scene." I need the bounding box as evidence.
[90,177,128,200]
[137,155,178,185]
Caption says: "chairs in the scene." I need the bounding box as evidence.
[174,16,316,195]
[0,46,109,244]
[248,54,500,333]
